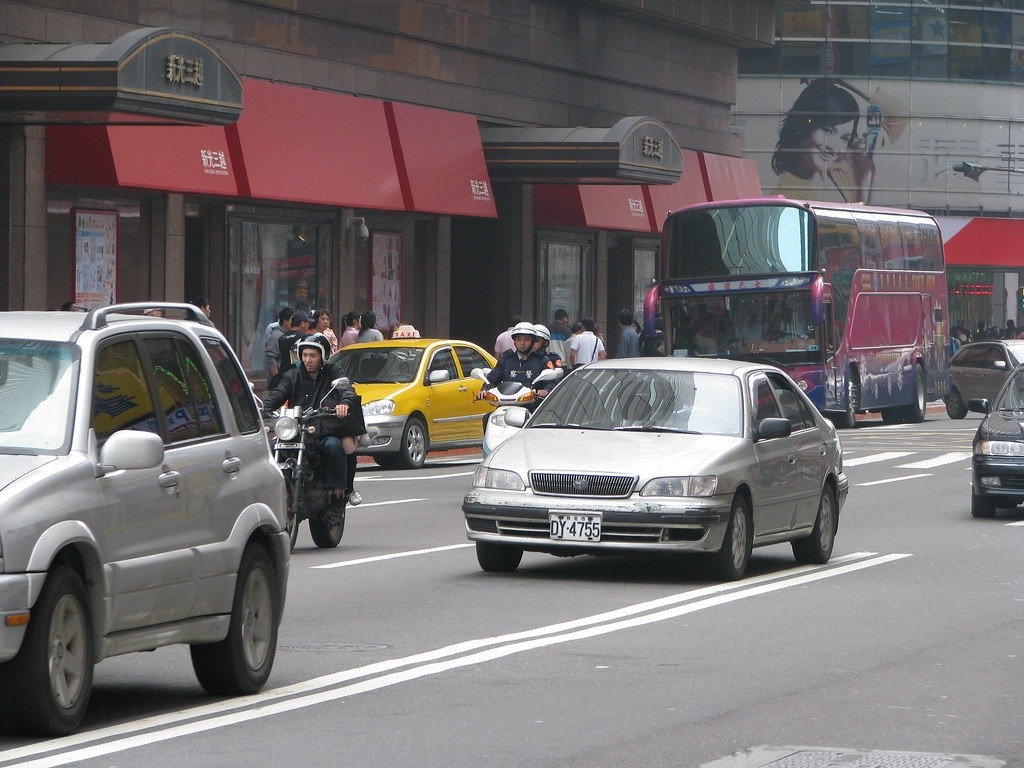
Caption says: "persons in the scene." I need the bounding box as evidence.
[476,322,558,436]
[493,315,522,360]
[357,311,387,372]
[265,300,338,393]
[770,76,911,205]
[144,308,162,317]
[260,332,362,517]
[61,302,76,309]
[950,318,1024,357]
[339,312,362,365]
[184,297,211,321]
[389,322,402,339]
[546,309,607,379]
[616,308,664,358]
[769,308,809,342]
[678,303,720,354]
[531,324,561,370]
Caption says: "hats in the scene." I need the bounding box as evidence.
[289,311,316,325]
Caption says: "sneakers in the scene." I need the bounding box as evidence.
[345,490,361,506]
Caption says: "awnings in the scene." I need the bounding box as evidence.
[44,75,498,217]
[533,149,764,233]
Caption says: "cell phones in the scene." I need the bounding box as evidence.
[865,105,882,158]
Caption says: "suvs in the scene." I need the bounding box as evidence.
[0,300,292,737]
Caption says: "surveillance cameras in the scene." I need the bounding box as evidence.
[357,224,370,242]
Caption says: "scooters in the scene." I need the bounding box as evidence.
[467,365,563,461]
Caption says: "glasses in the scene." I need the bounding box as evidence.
[534,337,542,343]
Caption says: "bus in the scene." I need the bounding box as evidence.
[644,194,951,429]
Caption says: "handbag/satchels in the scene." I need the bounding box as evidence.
[334,396,368,438]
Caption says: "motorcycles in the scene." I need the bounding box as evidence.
[247,376,359,553]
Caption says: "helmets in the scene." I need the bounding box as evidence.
[533,324,550,341]
[296,332,331,362]
[511,321,537,339]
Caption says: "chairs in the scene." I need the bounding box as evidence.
[360,359,389,380]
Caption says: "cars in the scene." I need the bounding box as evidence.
[970,363,1024,518]
[277,325,506,470]
[462,356,850,583]
[942,339,1024,420]
